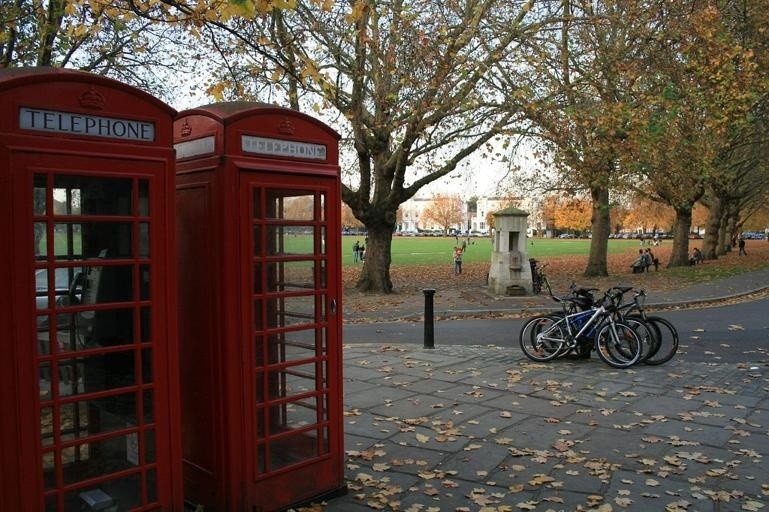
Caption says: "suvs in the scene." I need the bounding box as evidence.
[35,261,87,378]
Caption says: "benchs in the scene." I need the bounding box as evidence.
[689,256,705,265]
[631,261,662,273]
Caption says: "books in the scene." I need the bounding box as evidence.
[79,487,113,510]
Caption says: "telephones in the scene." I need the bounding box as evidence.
[66,246,111,323]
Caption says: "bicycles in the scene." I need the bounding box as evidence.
[520,283,681,370]
[530,257,554,296]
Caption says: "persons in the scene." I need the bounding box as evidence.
[352,236,368,265]
[453,247,463,276]
[687,247,703,266]
[452,236,471,260]
[640,249,652,272]
[647,248,654,260]
[744,231,763,240]
[629,248,645,273]
[738,238,747,257]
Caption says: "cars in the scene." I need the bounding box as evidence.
[740,231,765,239]
[687,232,699,239]
[343,229,368,236]
[392,227,489,237]
[559,233,592,239]
[608,231,675,239]
